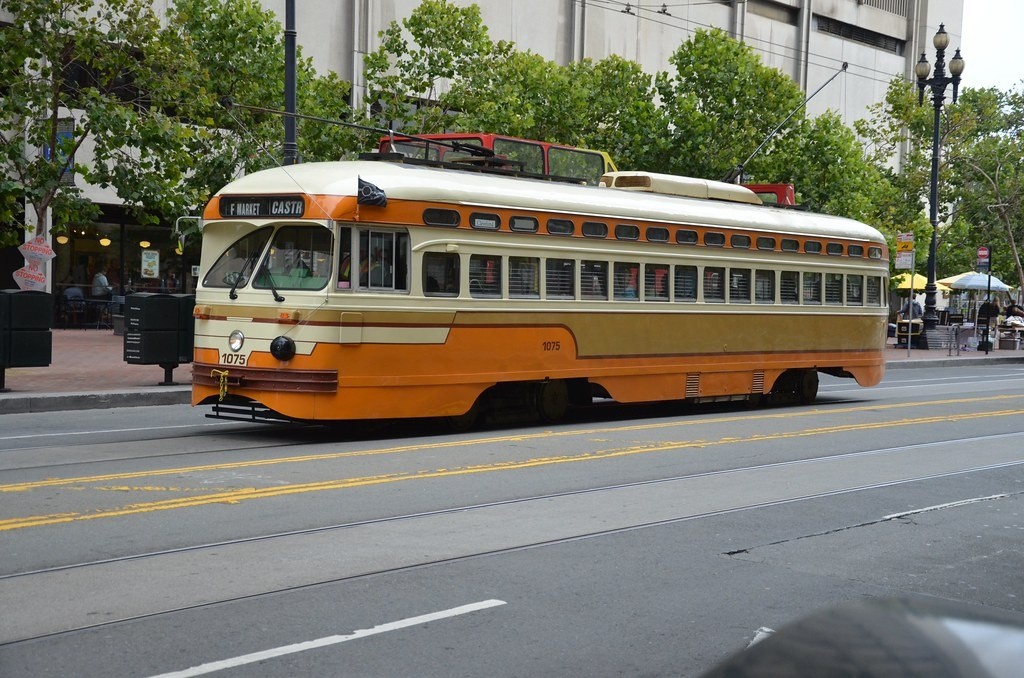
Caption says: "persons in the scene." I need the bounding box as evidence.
[339,239,386,286]
[897,293,923,319]
[1003,299,1024,318]
[546,263,857,302]
[989,297,1001,318]
[92,266,113,301]
[59,277,85,320]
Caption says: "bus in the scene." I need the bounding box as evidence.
[168,131,889,429]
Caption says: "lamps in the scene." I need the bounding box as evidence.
[98,237,112,250]
[138,240,153,248]
[55,235,71,244]
[173,247,186,257]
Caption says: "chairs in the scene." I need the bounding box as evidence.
[66,296,86,330]
[92,284,113,319]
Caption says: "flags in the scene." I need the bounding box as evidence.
[358,179,387,207]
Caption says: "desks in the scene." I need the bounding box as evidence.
[65,298,117,333]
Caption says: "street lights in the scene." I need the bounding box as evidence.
[915,22,965,332]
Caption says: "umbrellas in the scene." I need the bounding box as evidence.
[891,272,952,318]
[935,270,1014,322]
[952,272,1009,308]
